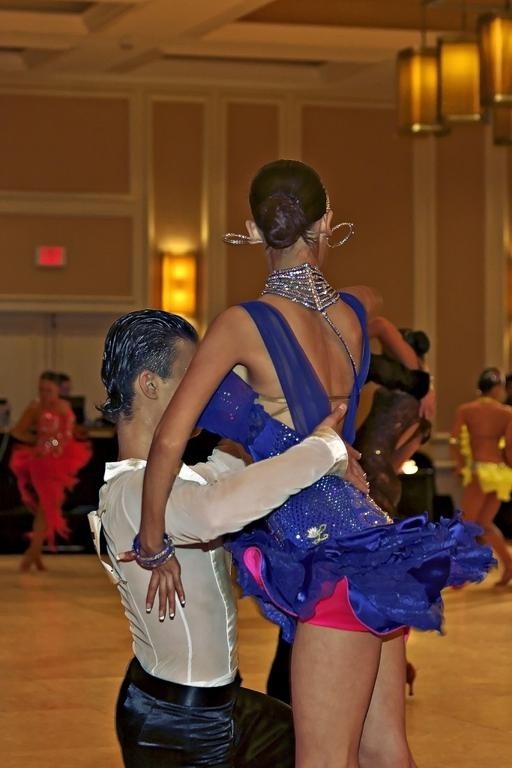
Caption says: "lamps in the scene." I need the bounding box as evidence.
[394,0,511,138]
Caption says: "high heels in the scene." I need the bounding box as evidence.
[405,661,416,697]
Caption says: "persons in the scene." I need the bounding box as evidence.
[97,305,369,764]
[2,373,248,572]
[352,318,511,587]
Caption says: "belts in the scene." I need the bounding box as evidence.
[128,659,242,710]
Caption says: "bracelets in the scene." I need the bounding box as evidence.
[127,524,174,574]
[137,157,416,767]
